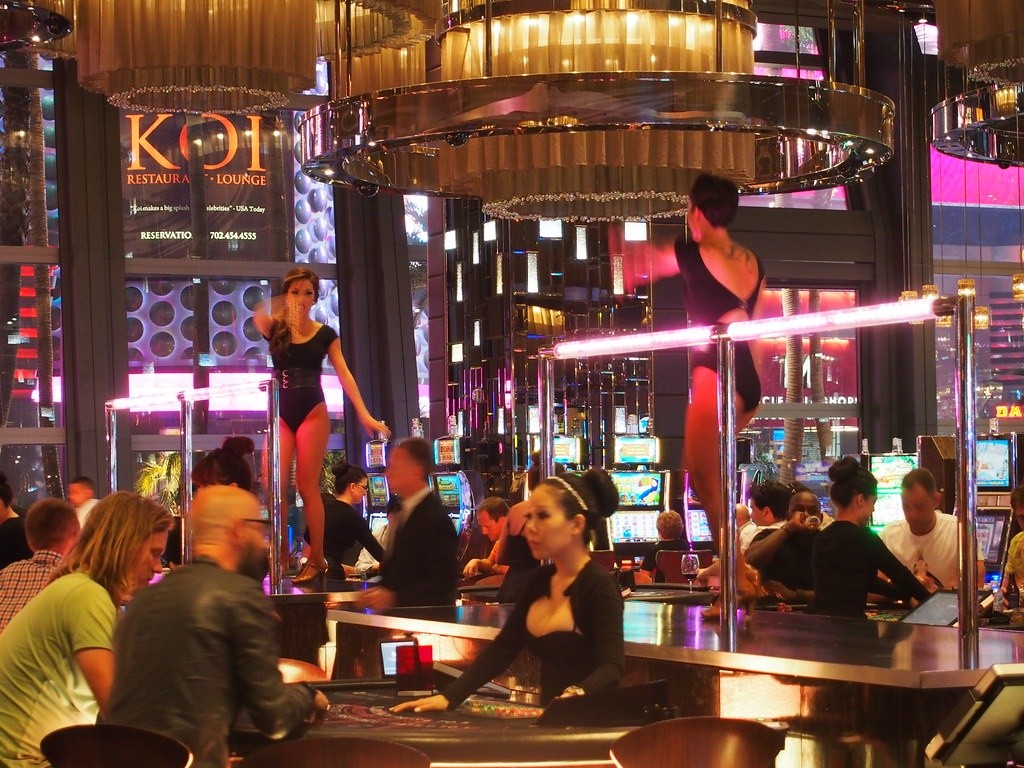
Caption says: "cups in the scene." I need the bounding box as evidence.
[1011,608,1024,624]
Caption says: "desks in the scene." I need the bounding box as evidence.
[228,675,645,768]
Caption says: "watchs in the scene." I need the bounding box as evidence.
[564,686,584,695]
[302,681,318,699]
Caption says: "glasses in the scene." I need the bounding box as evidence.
[246,519,271,527]
[358,485,369,492]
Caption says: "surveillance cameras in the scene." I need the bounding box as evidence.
[446,133,467,148]
[837,165,858,178]
[353,180,379,198]
[998,161,1010,169]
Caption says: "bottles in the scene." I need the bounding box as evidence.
[1019,583,1024,606]
[992,583,1002,612]
[1006,573,1019,609]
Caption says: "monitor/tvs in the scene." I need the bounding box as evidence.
[611,508,661,543]
[925,663,1024,768]
[871,493,906,526]
[370,517,389,533]
[449,517,462,535]
[686,508,713,543]
[898,588,993,626]
[976,514,1006,562]
[379,637,418,678]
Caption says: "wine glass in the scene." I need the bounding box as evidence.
[681,554,700,594]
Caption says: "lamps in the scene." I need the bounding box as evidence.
[0,0,1024,225]
[726,299,959,341]
[554,326,722,360]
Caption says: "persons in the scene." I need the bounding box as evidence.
[637,511,688,576]
[999,485,1024,610]
[191,436,255,490]
[0,491,174,768]
[303,460,385,580]
[0,470,33,571]
[697,468,985,617]
[463,498,510,578]
[390,470,623,713]
[0,498,80,633]
[360,438,459,609]
[675,173,766,621]
[253,267,392,583]
[105,487,329,768]
[495,454,565,603]
[69,477,99,529]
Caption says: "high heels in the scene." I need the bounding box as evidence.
[291,559,329,585]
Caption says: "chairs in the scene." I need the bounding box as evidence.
[608,716,780,768]
[41,659,431,768]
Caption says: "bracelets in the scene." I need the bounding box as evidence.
[794,588,804,602]
[781,526,791,534]
